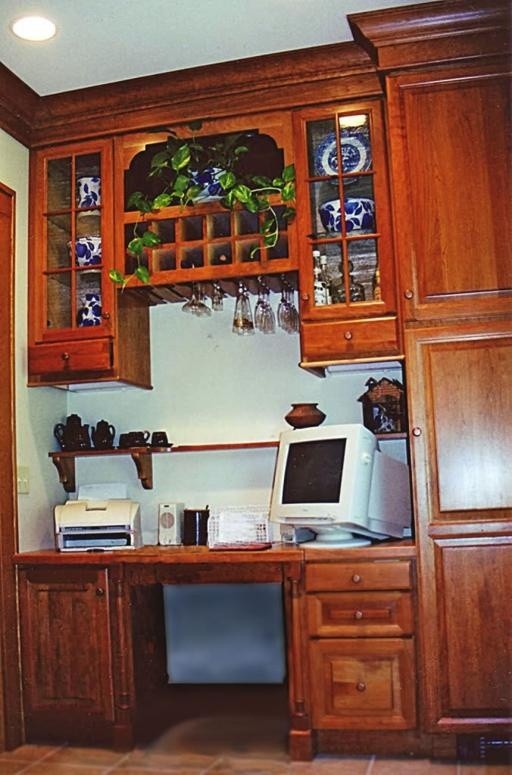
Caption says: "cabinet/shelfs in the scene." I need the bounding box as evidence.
[346,0,512,323]
[292,103,402,376]
[117,109,298,306]
[402,318,512,760]
[28,140,155,391]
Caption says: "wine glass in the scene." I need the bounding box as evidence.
[183,273,299,335]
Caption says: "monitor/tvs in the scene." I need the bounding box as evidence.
[269,424,412,548]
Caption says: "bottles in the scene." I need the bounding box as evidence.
[79,294,102,326]
[312,250,381,306]
[284,402,326,430]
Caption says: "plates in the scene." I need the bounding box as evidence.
[314,131,373,186]
[150,443,173,447]
[308,229,375,239]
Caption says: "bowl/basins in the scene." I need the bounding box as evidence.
[66,238,102,275]
[318,197,375,231]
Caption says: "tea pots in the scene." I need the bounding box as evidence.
[90,419,116,448]
[53,413,91,451]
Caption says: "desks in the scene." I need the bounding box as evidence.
[11,537,415,761]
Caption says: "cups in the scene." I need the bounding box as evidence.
[75,177,100,222]
[119,431,150,446]
[151,432,168,443]
[183,509,208,546]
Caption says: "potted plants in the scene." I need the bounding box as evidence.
[114,120,293,287]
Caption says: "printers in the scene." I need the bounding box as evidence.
[54,482,145,552]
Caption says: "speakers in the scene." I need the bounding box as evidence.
[158,502,185,545]
